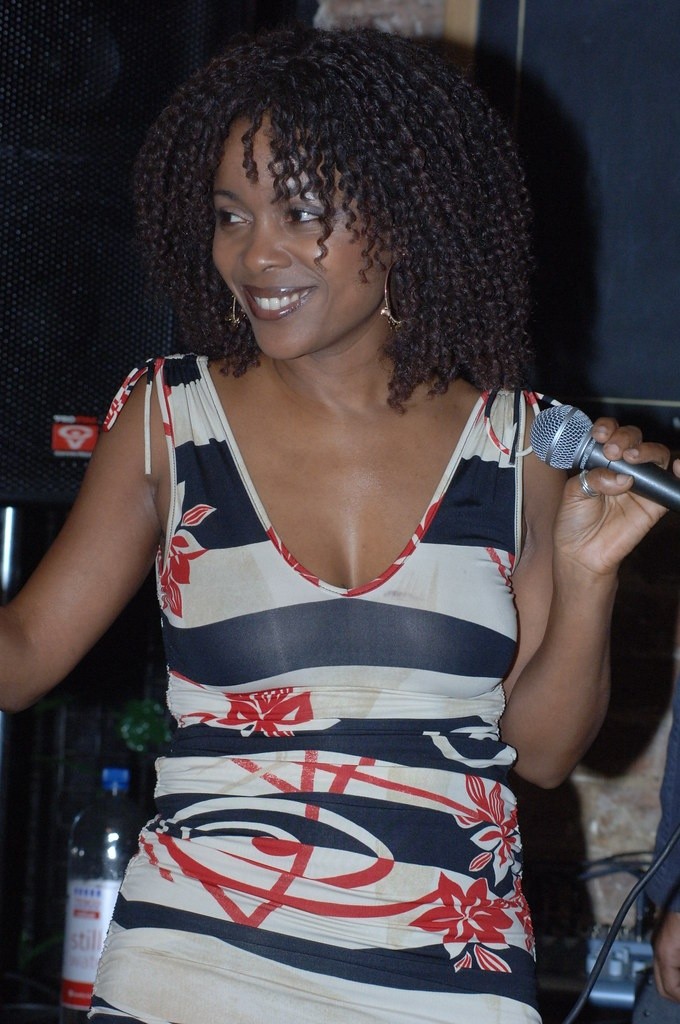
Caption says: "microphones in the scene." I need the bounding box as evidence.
[529,403,680,515]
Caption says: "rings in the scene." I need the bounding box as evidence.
[579,468,599,498]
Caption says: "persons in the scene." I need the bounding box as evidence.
[1,25,680,1022]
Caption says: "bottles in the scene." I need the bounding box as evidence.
[54,768,150,1023]
